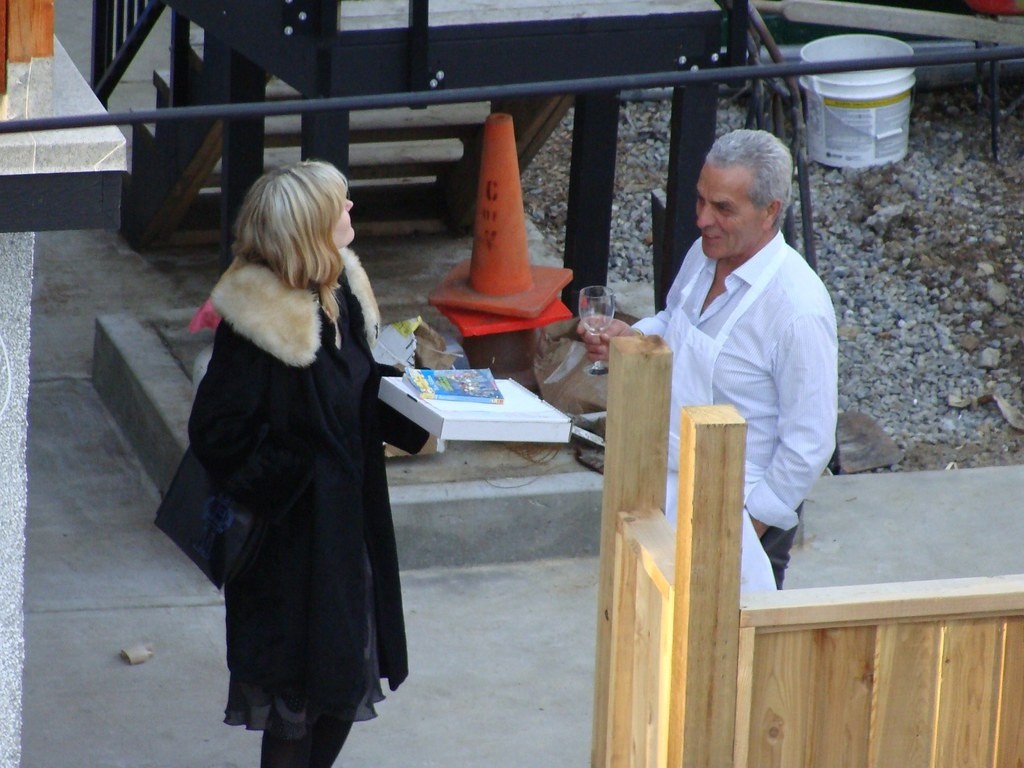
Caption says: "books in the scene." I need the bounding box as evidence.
[403,366,504,404]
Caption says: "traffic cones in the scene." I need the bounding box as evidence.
[426,112,575,337]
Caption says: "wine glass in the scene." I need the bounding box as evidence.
[578,285,615,377]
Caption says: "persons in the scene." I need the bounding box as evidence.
[576,129,840,591]
[188,160,430,767]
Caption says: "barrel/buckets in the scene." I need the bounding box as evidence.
[799,34,917,169]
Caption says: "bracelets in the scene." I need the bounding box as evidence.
[634,329,643,336]
